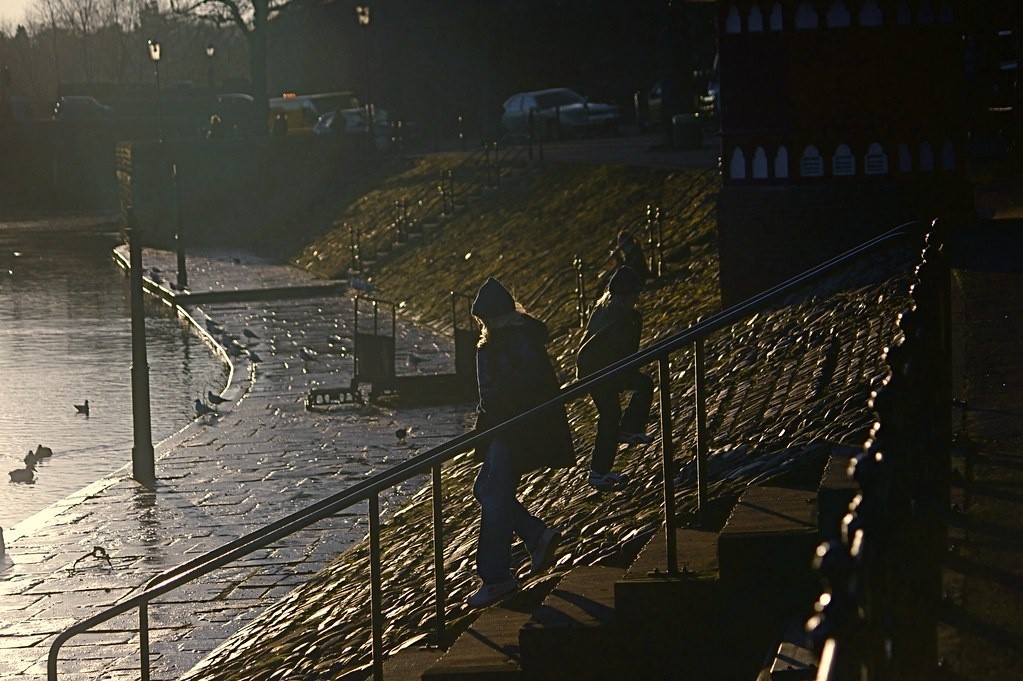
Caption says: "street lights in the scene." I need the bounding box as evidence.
[205,40,216,115]
[355,3,377,153]
[147,39,167,144]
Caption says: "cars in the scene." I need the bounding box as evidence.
[500,87,619,137]
[221,93,254,113]
[313,104,427,154]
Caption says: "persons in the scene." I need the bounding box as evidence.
[206,115,225,138]
[596,230,649,298]
[467,277,576,608]
[272,111,288,137]
[572,266,651,488]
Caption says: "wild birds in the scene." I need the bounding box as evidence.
[407,352,431,368]
[169,271,191,294]
[325,336,344,345]
[193,399,219,415]
[149,267,164,283]
[8,464,38,482]
[24,444,53,466]
[229,258,241,265]
[73,399,89,413]
[298,349,318,366]
[207,390,232,411]
[395,427,406,441]
[205,319,264,368]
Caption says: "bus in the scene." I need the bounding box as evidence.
[267,92,361,153]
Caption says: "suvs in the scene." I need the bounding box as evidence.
[63,95,114,120]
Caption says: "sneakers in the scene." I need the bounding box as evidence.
[618,429,653,444]
[468,577,517,607]
[530,529,561,576]
[588,470,622,486]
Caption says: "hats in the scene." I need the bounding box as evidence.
[609,265,644,295]
[471,277,516,319]
